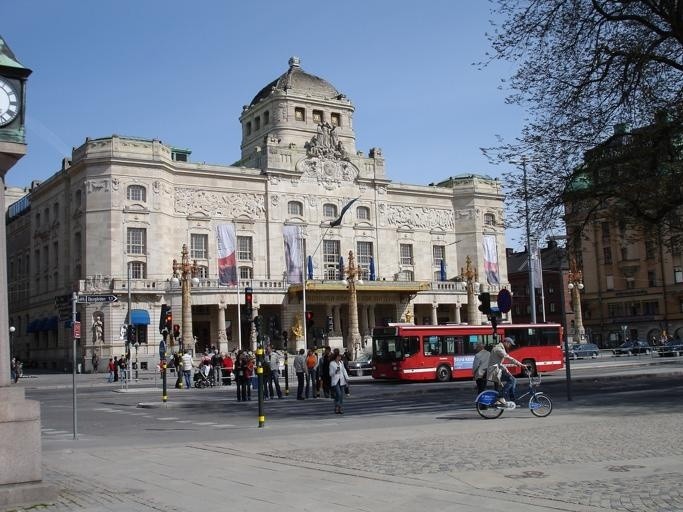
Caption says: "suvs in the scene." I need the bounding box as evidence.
[561,343,600,360]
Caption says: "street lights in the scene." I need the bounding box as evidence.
[339,250,364,360]
[9,325,17,363]
[459,255,481,325]
[169,242,201,356]
[567,260,585,343]
[620,323,628,342]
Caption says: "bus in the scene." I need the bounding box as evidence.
[372,321,563,383]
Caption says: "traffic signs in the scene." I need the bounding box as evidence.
[78,294,118,302]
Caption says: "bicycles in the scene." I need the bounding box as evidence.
[473,363,554,419]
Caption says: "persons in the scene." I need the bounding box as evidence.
[11,357,16,377]
[92,353,100,371]
[93,317,103,342]
[14,360,21,382]
[173,346,353,414]
[487,336,527,408]
[108,354,127,382]
[652,334,672,350]
[472,343,491,395]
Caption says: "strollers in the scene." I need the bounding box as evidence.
[192,366,213,390]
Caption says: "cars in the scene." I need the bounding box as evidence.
[657,340,683,357]
[344,352,372,379]
[611,340,651,356]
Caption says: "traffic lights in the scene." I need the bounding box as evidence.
[305,312,313,329]
[323,314,333,333]
[138,326,143,344]
[173,324,179,336]
[477,293,489,314]
[159,303,169,330]
[167,312,172,330]
[244,287,252,319]
[131,328,136,343]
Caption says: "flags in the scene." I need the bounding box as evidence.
[529,241,543,288]
[217,224,237,285]
[483,234,500,283]
[329,198,360,227]
[282,223,306,284]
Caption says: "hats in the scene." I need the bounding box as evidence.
[504,337,516,346]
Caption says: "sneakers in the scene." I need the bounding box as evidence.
[496,397,506,405]
